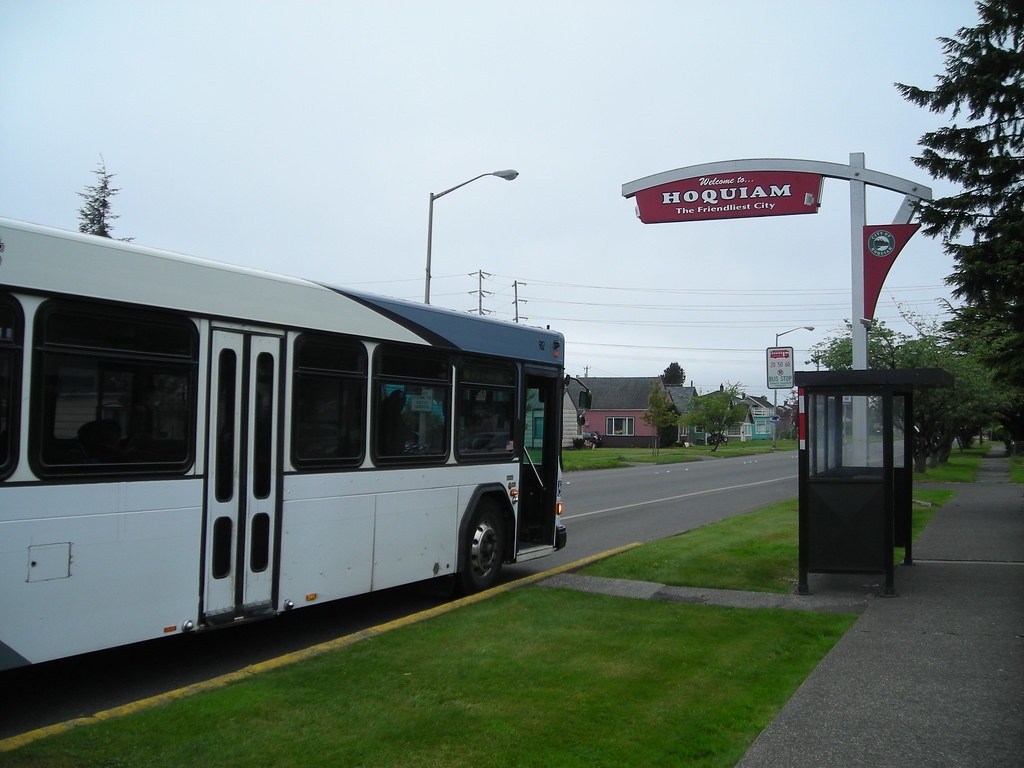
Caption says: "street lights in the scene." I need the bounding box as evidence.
[425,169,519,304]
[771,326,815,450]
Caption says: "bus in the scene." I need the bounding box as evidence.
[0,219,592,673]
